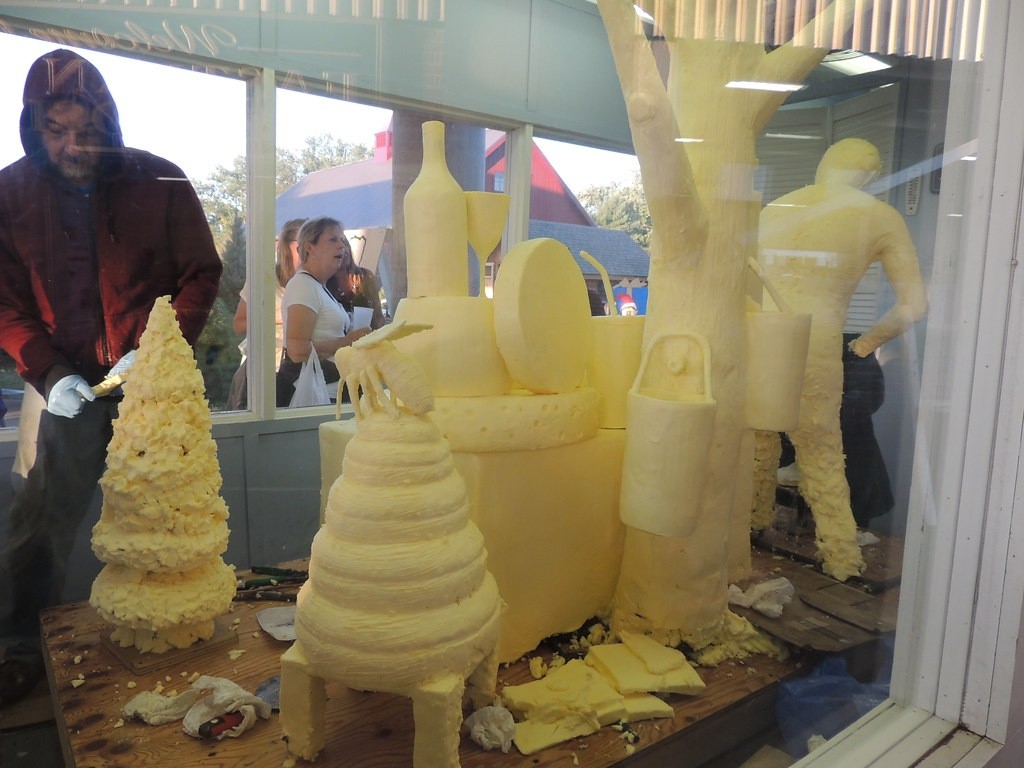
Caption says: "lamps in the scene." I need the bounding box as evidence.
[818,47,899,79]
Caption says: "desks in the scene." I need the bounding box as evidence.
[39,558,811,768]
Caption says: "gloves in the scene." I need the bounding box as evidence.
[47,374,94,419]
[104,350,140,397]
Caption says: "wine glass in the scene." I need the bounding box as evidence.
[456,192,511,298]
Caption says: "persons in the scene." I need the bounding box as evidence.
[748,138,930,596]
[0,48,223,708]
[224,217,388,411]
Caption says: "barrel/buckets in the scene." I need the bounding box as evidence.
[741,256,812,435]
[621,328,718,538]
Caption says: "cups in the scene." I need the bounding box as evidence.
[353,306,374,334]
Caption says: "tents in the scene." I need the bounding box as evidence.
[275,158,392,237]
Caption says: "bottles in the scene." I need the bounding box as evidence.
[399,120,471,299]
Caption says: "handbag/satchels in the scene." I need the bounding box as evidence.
[289,344,331,407]
